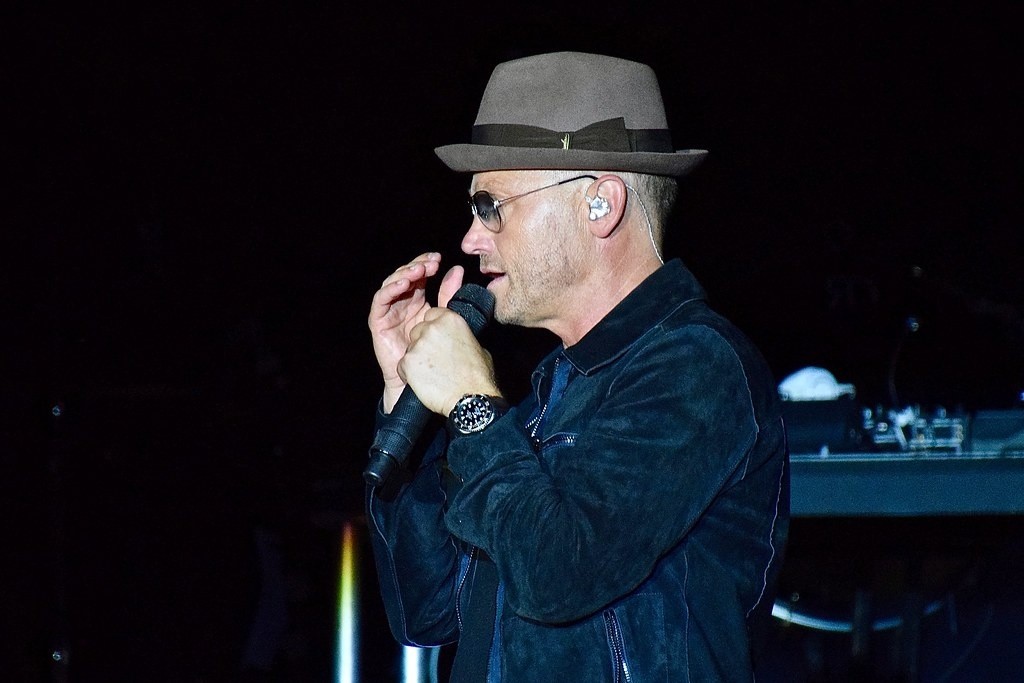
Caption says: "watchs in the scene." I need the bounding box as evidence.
[445,392,509,435]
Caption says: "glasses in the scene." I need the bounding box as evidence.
[468,175,598,233]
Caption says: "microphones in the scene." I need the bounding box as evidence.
[362,282,496,488]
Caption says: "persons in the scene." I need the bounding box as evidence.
[362,51,791,682]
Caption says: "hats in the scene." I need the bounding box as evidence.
[433,52,710,175]
